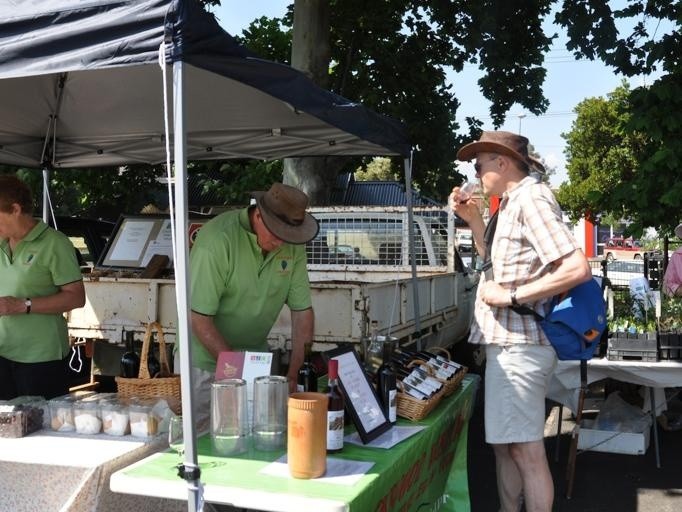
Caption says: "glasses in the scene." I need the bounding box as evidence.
[475,157,497,173]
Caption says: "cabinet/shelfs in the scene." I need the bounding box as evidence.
[546,358,682,468]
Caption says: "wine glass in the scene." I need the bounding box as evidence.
[168,416,185,472]
[443,179,477,217]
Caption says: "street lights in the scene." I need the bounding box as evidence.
[517,114,527,134]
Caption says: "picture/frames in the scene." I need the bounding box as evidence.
[321,344,392,445]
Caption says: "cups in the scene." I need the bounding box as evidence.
[285,391,330,478]
[208,376,250,456]
[253,375,292,451]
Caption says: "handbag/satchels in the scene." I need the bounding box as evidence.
[540,278,606,360]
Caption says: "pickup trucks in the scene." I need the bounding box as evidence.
[62,206,486,379]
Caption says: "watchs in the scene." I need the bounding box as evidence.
[25,297,32,314]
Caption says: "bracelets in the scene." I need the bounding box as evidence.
[510,288,517,306]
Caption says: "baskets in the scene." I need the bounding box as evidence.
[396,346,468,420]
[115,322,182,416]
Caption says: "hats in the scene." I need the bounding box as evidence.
[243,182,320,244]
[456,131,546,175]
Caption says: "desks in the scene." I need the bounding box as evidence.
[0,397,210,511]
[109,374,480,511]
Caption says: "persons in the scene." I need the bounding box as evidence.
[446,130,594,512]
[662,220,682,299]
[171,180,320,391]
[1,171,86,399]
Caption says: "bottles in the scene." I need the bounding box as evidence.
[322,358,347,453]
[365,319,465,426]
[120,329,141,379]
[142,331,161,379]
[297,340,319,391]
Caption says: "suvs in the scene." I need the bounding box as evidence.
[603,236,662,265]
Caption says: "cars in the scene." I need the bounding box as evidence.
[455,231,475,253]
[35,214,118,277]
